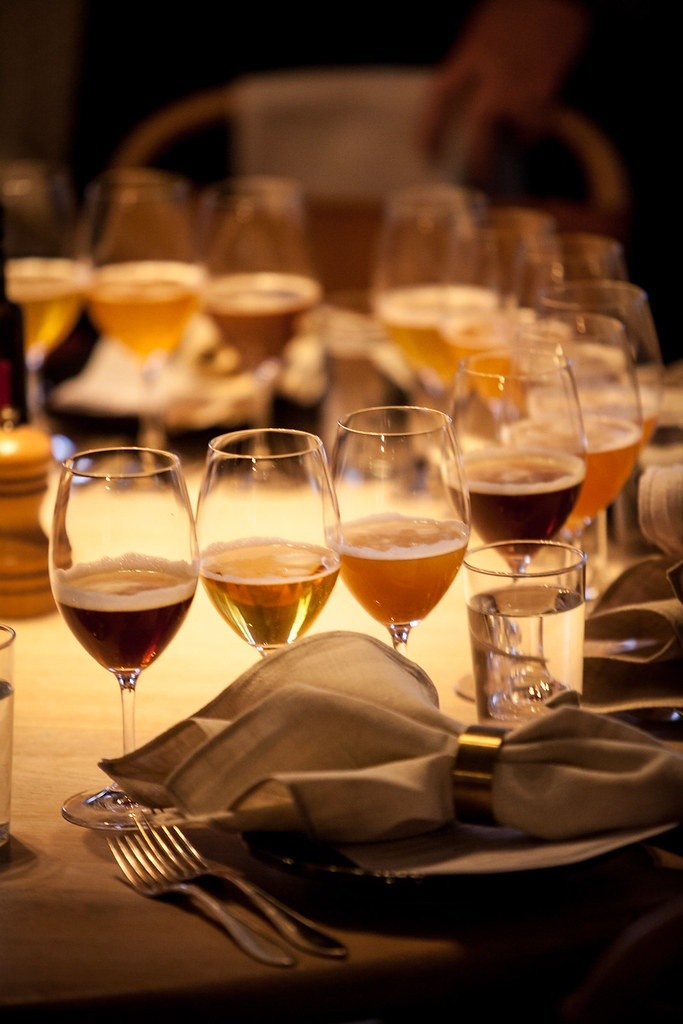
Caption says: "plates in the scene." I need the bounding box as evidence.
[235,820,664,908]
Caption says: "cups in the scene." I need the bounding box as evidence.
[0,623,17,870]
[460,540,585,726]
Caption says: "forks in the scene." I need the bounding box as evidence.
[104,831,299,967]
[133,810,350,959]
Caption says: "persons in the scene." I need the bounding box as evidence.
[60,4,682,366]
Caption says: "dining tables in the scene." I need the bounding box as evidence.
[0,369,683,1012]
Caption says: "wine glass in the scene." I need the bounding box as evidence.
[198,174,324,510]
[436,209,575,530]
[521,311,645,691]
[368,178,502,528]
[48,447,237,828]
[512,230,640,587]
[76,165,204,513]
[331,405,472,664]
[193,428,345,664]
[536,274,663,624]
[439,346,585,699]
[1,159,92,491]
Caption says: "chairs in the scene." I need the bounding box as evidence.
[119,67,618,292]
